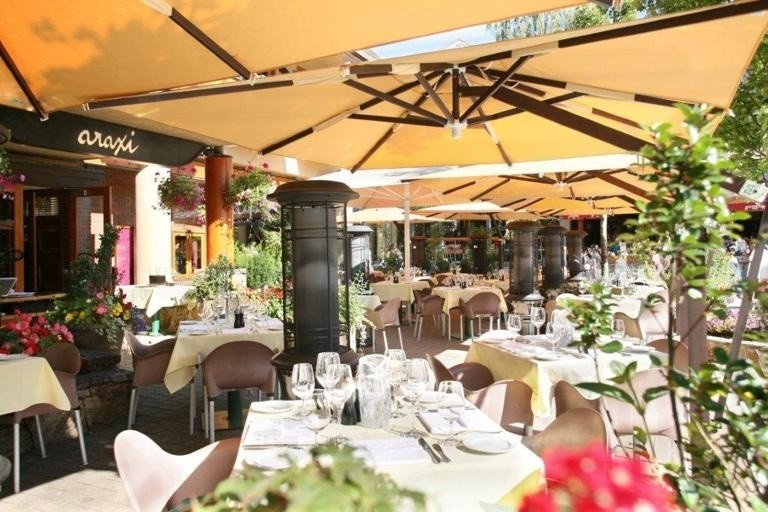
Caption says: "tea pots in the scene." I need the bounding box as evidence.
[355,352,390,429]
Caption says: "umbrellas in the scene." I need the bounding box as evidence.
[1,0,612,116]
[59,0,768,173]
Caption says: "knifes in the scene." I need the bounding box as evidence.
[416,435,449,464]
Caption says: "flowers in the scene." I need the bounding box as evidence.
[221,163,285,225]
[151,166,208,226]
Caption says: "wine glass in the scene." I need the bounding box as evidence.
[445,265,506,289]
[609,319,625,354]
[291,349,465,456]
[387,265,424,283]
[505,299,572,356]
[195,299,223,335]
[577,274,640,298]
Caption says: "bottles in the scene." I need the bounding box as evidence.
[232,307,245,328]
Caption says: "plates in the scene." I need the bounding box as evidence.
[245,449,312,470]
[249,401,293,413]
[406,390,448,401]
[463,433,512,455]
[478,328,508,344]
[533,350,564,361]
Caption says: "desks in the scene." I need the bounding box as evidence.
[1,353,64,437]
